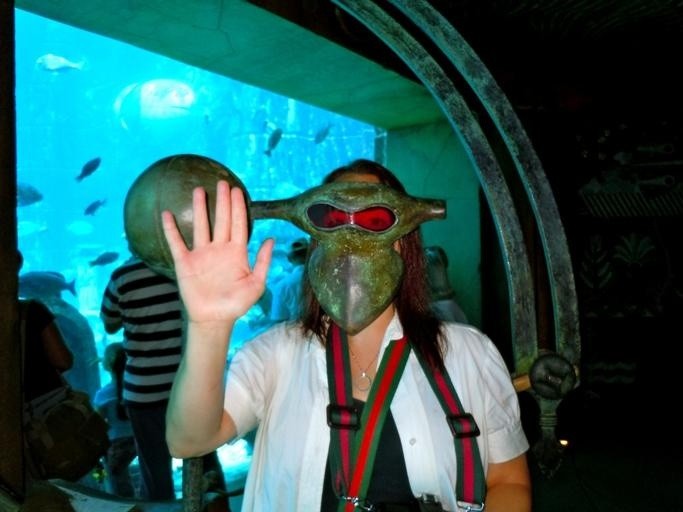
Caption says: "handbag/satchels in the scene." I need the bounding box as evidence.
[24,388,110,482]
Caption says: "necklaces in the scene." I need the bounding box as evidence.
[346,343,380,392]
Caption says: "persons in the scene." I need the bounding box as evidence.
[98,257,232,511]
[16,244,74,491]
[262,234,320,324]
[91,340,139,501]
[157,160,539,512]
